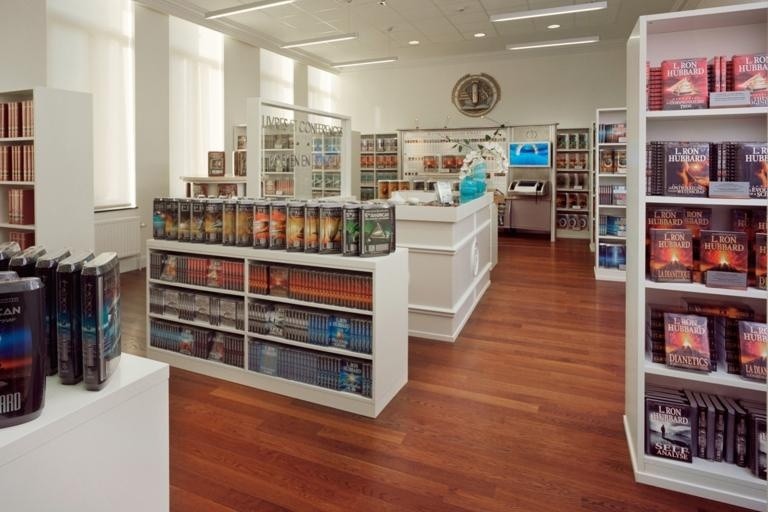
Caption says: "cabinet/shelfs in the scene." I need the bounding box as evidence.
[556,107,627,283]
[0,87,95,258]
[145,240,408,419]
[623,2,768,511]
[232,124,397,200]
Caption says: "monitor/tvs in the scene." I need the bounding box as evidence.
[508,141,551,167]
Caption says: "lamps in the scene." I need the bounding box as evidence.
[505,34,600,52]
[490,1,607,24]
[203,0,297,20]
[279,32,359,50]
[332,56,398,68]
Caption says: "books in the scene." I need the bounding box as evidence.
[218,183,237,197]
[646,203,768,291]
[553,123,626,270]
[208,152,225,176]
[263,129,397,201]
[233,136,247,176]
[423,154,466,173]
[646,52,768,111]
[0,100,34,252]
[646,139,768,199]
[644,296,768,482]
[148,249,373,399]
[192,184,209,196]
